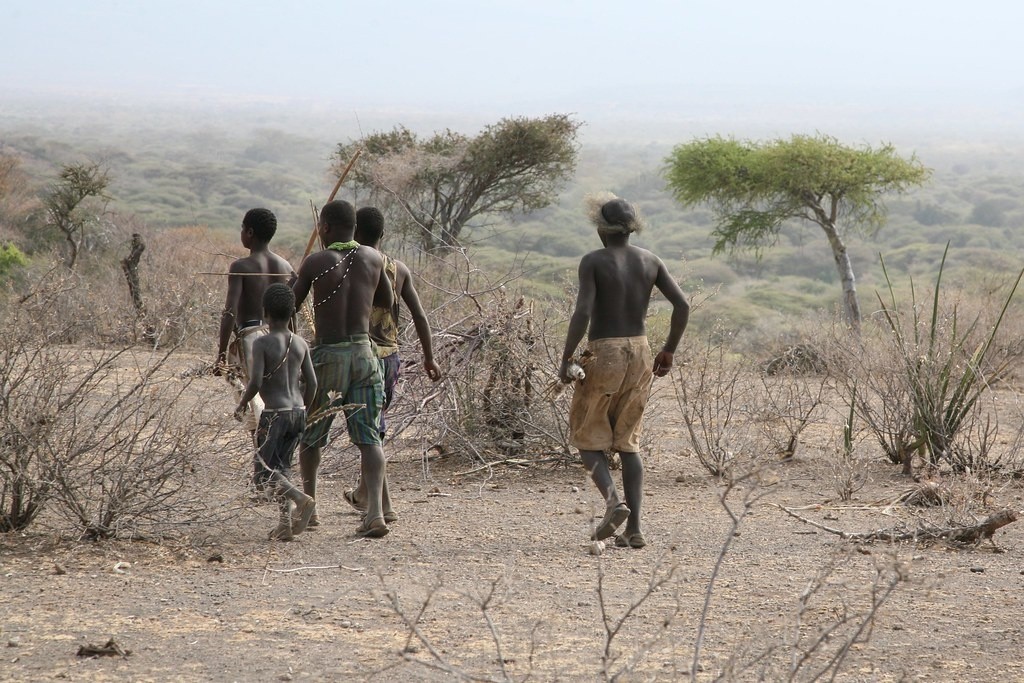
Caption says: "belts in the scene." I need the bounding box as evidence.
[263,406,306,412]
[314,334,378,357]
[240,320,266,330]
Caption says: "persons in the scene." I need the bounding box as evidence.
[291,200,394,537]
[557,198,690,547]
[233,283,318,542]
[212,208,294,491]
[343,207,442,521]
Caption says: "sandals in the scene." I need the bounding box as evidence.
[591,502,631,540]
[292,496,317,536]
[384,511,398,523]
[342,489,366,511]
[267,522,294,542]
[355,515,390,538]
[615,533,647,547]
